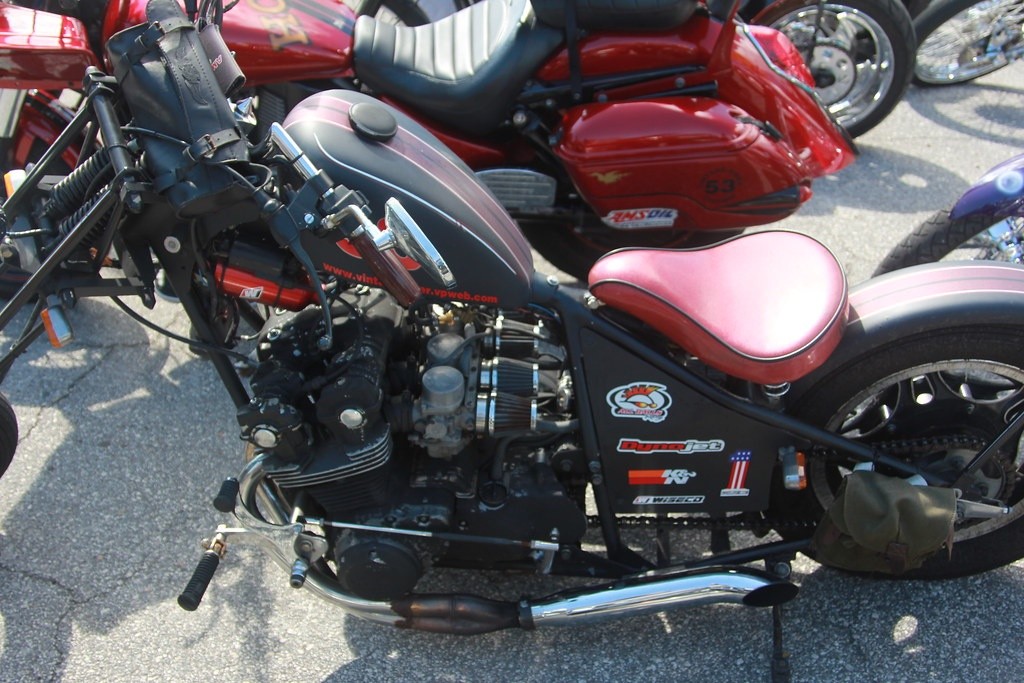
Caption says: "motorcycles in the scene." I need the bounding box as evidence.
[0,0,1024,683]
